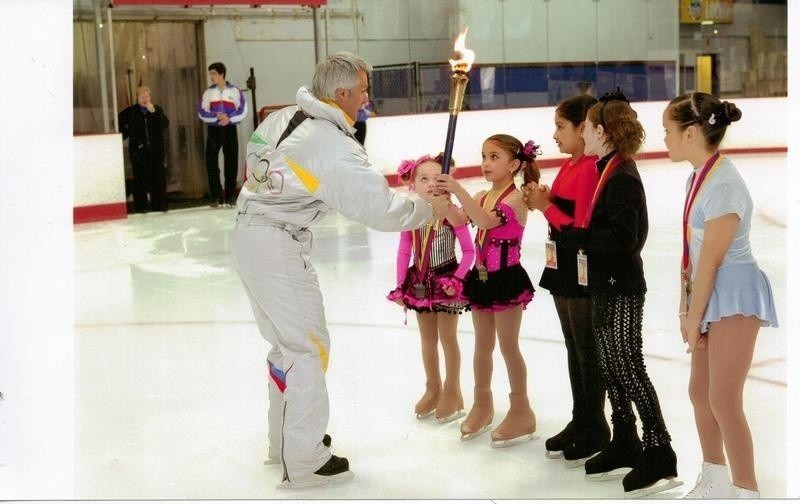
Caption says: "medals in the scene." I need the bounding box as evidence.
[684,279,691,298]
[478,266,489,284]
[415,283,427,300]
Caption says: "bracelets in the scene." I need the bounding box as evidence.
[678,310,689,318]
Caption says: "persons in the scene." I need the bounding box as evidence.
[228,50,452,485]
[195,60,247,208]
[429,134,544,443]
[353,90,377,148]
[521,96,612,462]
[384,150,477,421]
[661,89,780,500]
[560,91,677,493]
[116,85,169,215]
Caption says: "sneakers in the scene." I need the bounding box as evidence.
[460,386,494,434]
[585,424,644,474]
[437,383,464,418]
[314,455,349,476]
[491,392,536,441]
[323,434,332,446]
[546,408,611,460]
[622,444,677,492]
[415,380,442,414]
[683,461,760,498]
